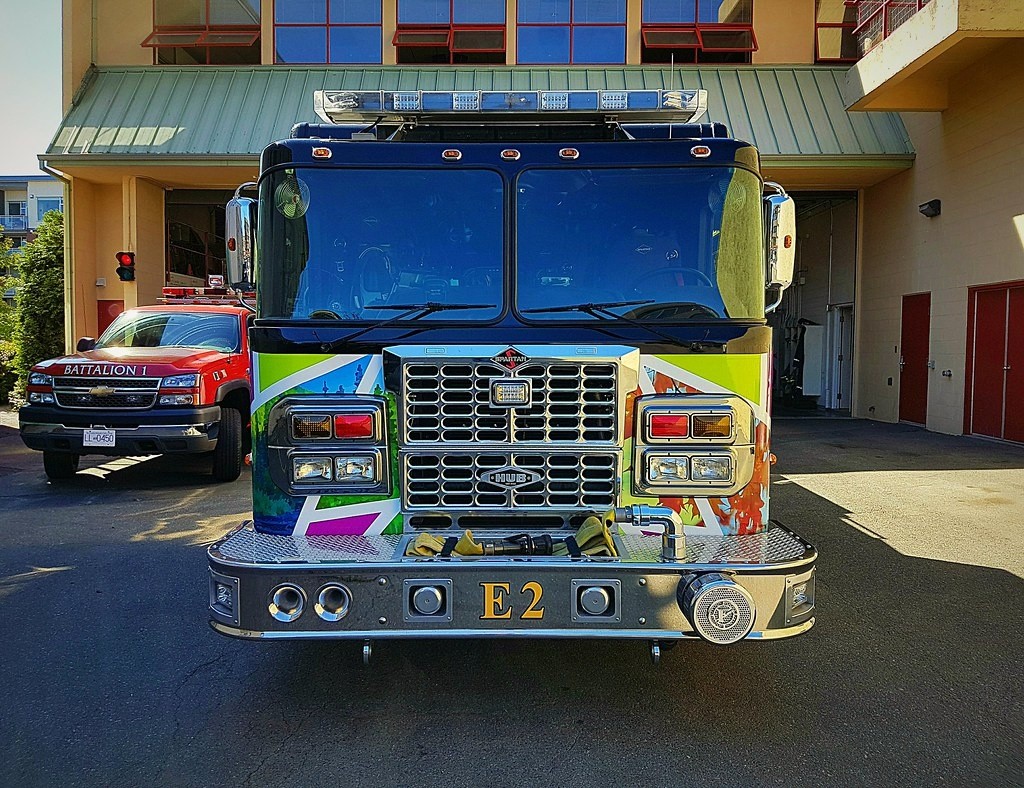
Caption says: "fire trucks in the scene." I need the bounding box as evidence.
[205,88,818,668]
[19,274,257,487]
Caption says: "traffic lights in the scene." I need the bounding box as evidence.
[115,251,135,281]
